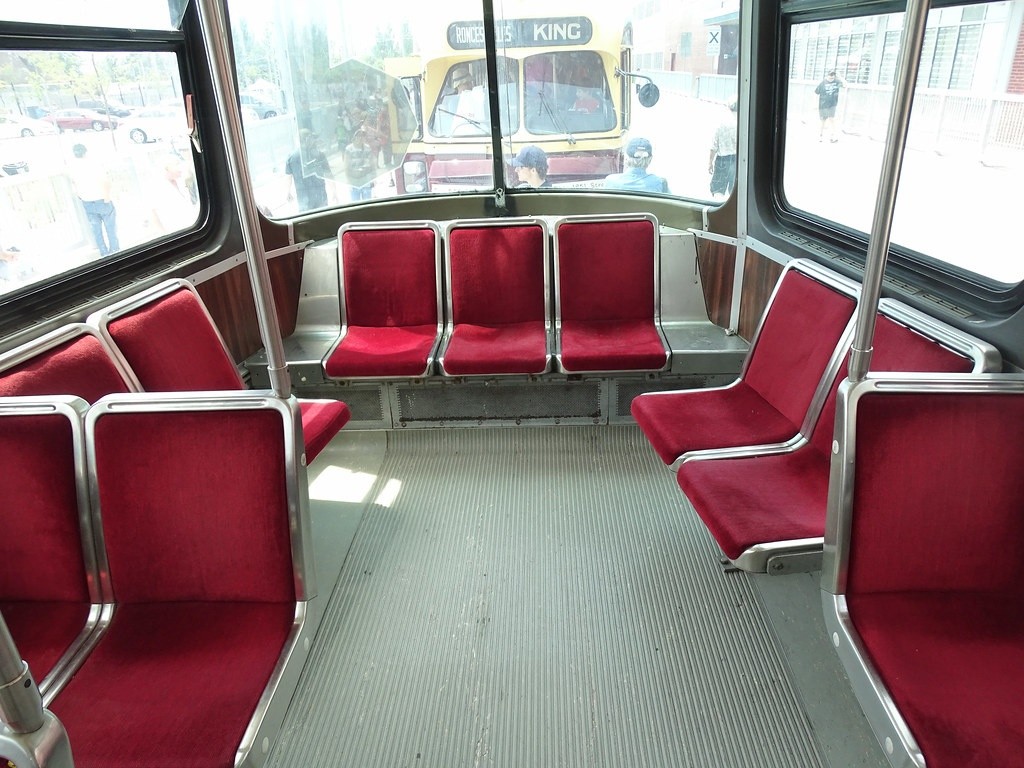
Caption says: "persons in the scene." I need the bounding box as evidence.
[503,145,553,189]
[602,138,671,195]
[569,77,600,114]
[707,94,738,203]
[72,144,120,258]
[378,99,394,169]
[452,69,474,95]
[343,129,377,202]
[813,69,843,143]
[359,113,381,151]
[285,129,335,214]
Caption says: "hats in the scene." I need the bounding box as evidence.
[508,146,546,168]
[627,138,652,158]
[452,68,473,89]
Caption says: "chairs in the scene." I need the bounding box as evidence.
[321,219,446,380]
[675,297,1003,575]
[88,278,350,467]
[820,370,1024,767]
[12,389,321,768]
[434,220,552,378]
[1,394,102,768]
[0,324,139,405]
[554,212,673,375]
[629,258,865,472]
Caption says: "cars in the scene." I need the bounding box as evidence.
[1,86,288,181]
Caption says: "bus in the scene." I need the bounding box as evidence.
[386,15,662,194]
